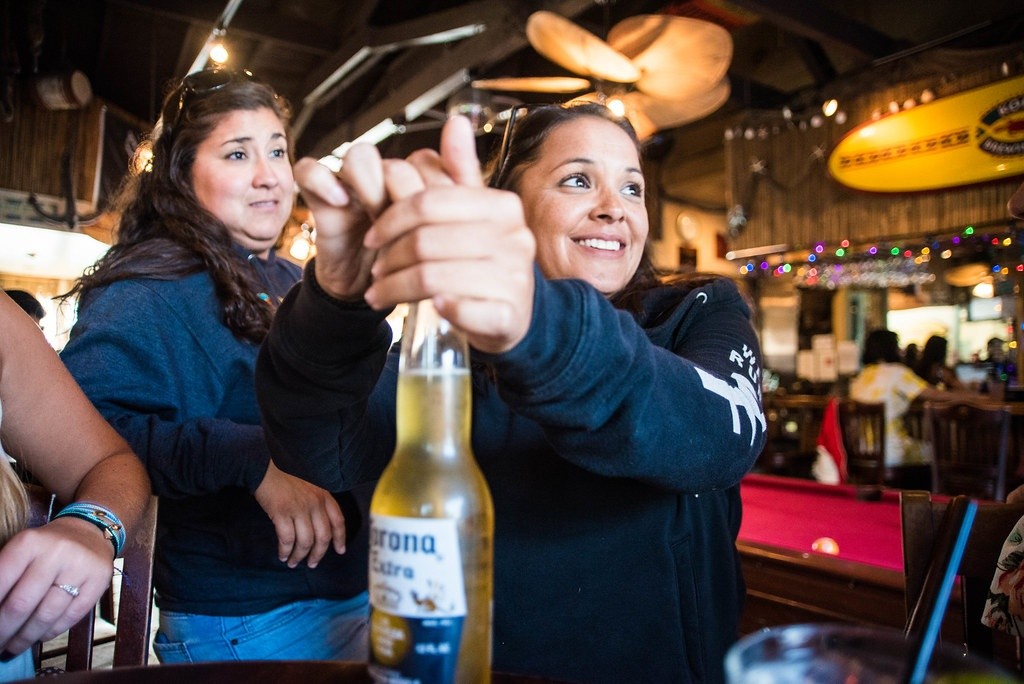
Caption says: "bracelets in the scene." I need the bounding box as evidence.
[47,495,126,561]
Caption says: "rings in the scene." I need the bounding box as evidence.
[51,582,79,598]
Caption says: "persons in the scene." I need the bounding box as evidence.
[898,336,1016,402]
[3,290,45,327]
[62,71,399,665]
[0,282,151,684]
[850,328,967,492]
[255,100,772,684]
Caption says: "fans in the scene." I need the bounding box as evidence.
[470,0,733,138]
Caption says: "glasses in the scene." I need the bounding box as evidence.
[496,98,622,182]
[169,66,266,167]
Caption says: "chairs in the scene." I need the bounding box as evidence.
[20,486,160,678]
[761,383,1015,503]
[898,486,1024,648]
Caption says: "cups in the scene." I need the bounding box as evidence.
[723,622,1017,684]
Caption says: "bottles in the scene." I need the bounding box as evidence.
[367,298,495,684]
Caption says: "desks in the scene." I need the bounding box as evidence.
[733,474,1018,649]
